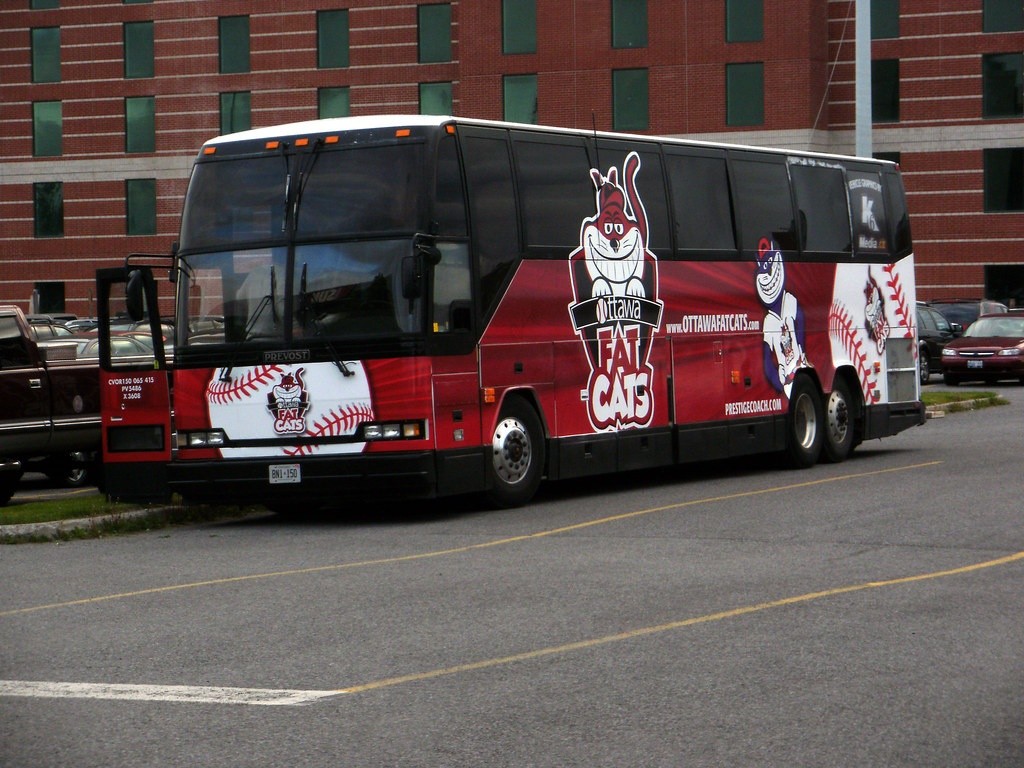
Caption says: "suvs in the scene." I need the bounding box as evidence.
[928,298,1010,340]
[915,301,955,386]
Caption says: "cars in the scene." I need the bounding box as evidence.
[940,312,1024,387]
[24,311,213,359]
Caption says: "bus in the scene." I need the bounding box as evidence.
[96,117,927,511]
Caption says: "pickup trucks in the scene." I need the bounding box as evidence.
[0,304,175,507]
